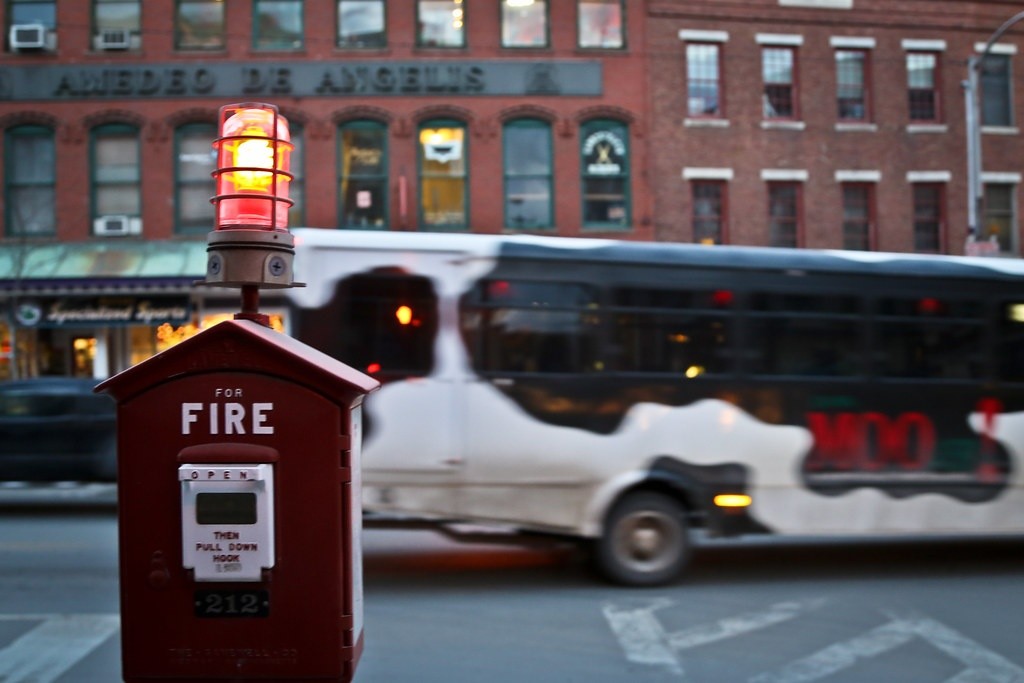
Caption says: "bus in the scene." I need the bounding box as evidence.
[195,226,1024,587]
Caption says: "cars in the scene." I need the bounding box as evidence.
[0,375,119,484]
